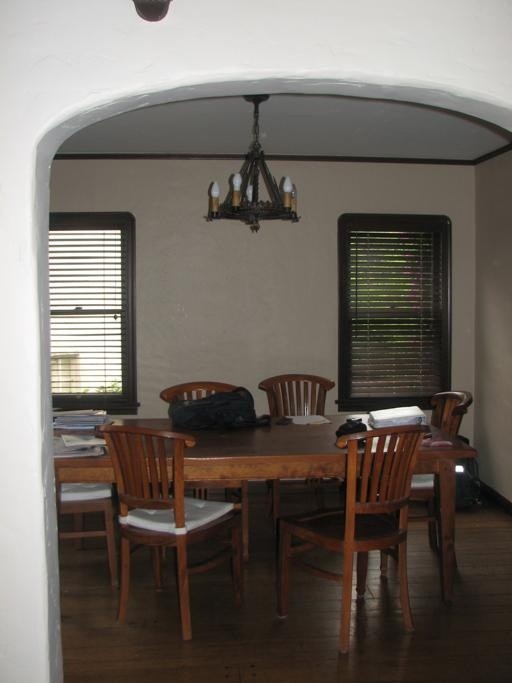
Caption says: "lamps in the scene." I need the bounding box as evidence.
[203,94,300,232]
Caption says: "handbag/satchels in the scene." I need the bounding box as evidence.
[167,386,275,434]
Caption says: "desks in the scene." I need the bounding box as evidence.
[55,410,478,604]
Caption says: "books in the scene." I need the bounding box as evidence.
[61,434,107,447]
[52,409,107,430]
[368,405,427,429]
[52,437,104,459]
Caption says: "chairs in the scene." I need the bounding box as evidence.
[97,424,245,640]
[369,391,473,577]
[160,382,237,503]
[58,486,117,595]
[258,372,339,527]
[274,425,430,655]
